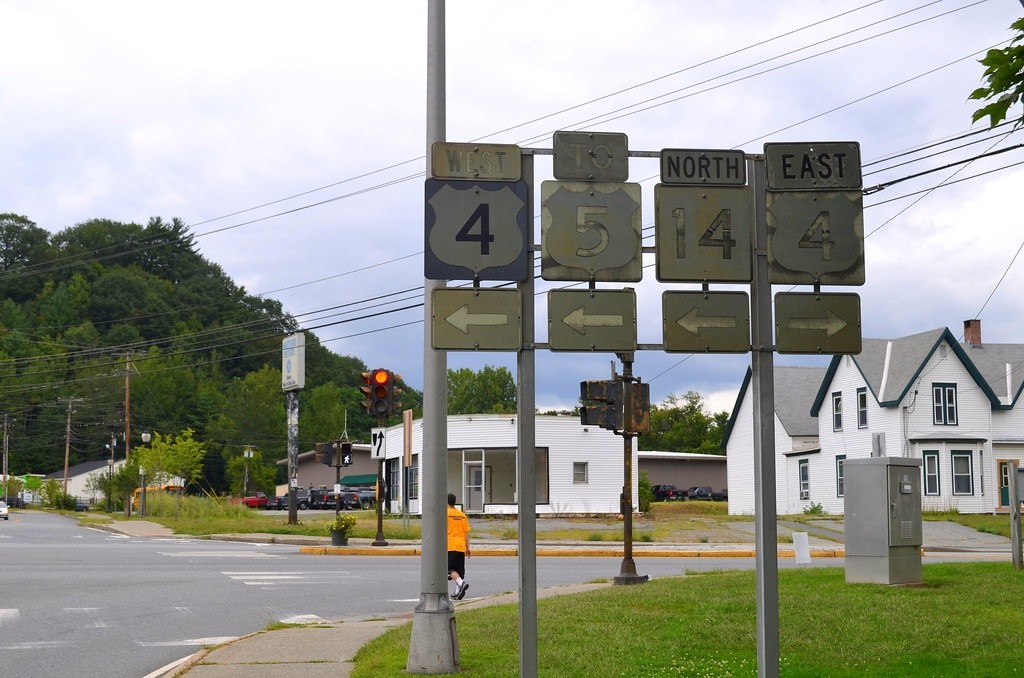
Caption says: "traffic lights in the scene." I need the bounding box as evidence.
[371,368,392,417]
[314,442,332,465]
[340,442,353,465]
[360,370,372,416]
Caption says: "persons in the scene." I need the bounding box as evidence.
[447,493,472,600]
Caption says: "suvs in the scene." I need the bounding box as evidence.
[687,487,713,501]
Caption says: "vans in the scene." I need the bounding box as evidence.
[0,496,24,508]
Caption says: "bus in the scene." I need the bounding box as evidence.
[134,485,187,508]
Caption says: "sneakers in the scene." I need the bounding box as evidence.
[450,591,459,598]
[456,581,469,601]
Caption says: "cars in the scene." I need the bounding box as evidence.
[0,501,10,520]
[266,496,284,510]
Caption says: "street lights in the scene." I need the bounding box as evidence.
[106,457,113,513]
[139,430,151,518]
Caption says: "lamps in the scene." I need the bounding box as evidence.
[510,419,514,424]
[584,428,588,432]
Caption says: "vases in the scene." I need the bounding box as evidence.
[331,532,348,546]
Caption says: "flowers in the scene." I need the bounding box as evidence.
[327,514,358,544]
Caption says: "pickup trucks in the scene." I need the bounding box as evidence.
[650,483,686,502]
[713,488,728,500]
[282,488,376,511]
